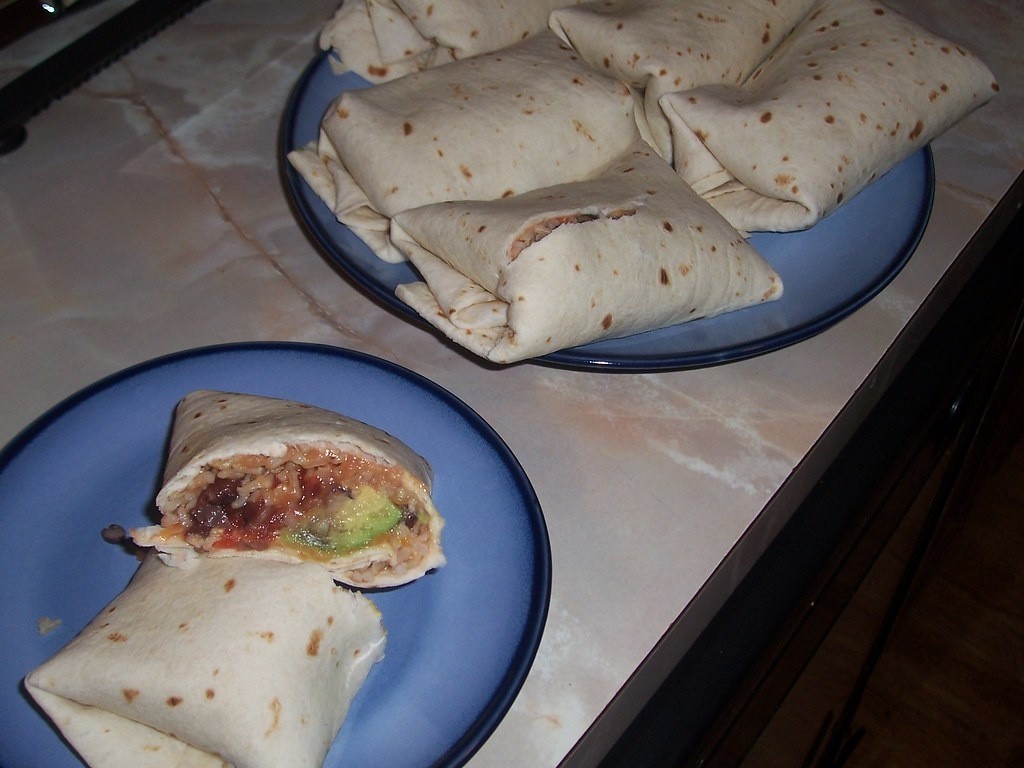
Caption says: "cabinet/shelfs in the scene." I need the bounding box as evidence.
[0,0,1024,768]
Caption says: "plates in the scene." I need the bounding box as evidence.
[0,341,552,768]
[277,45,936,373]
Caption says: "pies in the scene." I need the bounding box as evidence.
[25,544,387,767]
[127,391,446,591]
[284,0,1000,363]
